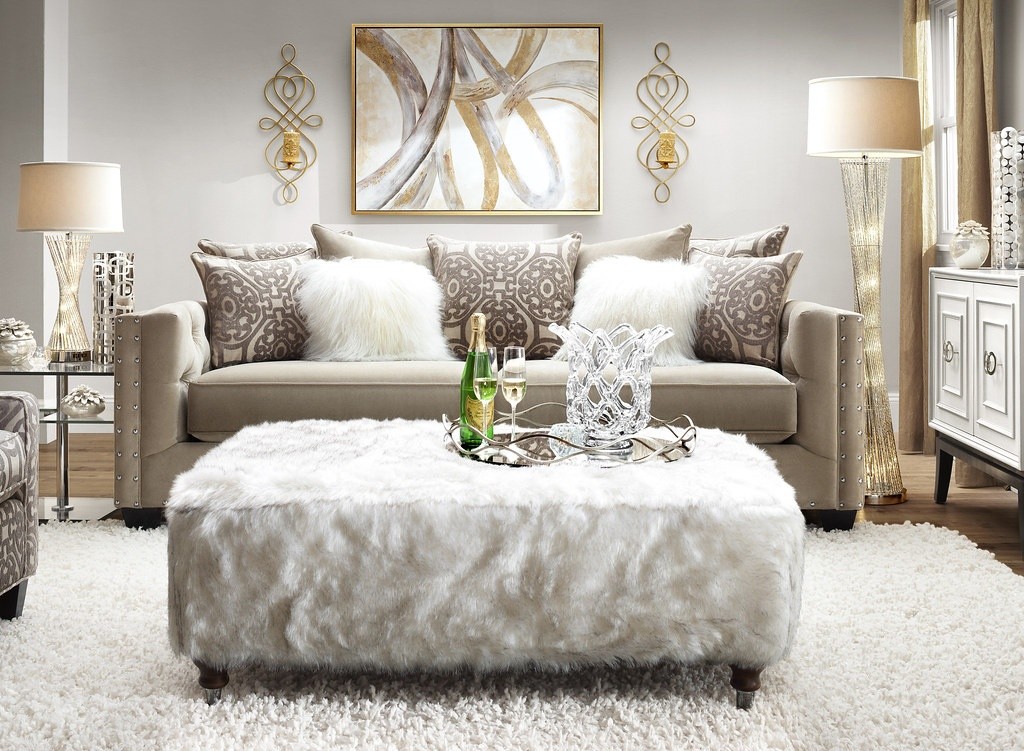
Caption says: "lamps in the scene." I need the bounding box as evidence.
[19,163,124,362]
[810,76,923,505]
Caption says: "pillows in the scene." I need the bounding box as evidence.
[549,255,707,367]
[688,247,804,371]
[198,239,309,261]
[574,223,692,292]
[311,223,432,274]
[688,224,789,261]
[191,248,316,368]
[296,255,460,361]
[427,231,583,360]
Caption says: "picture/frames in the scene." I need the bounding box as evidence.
[351,24,604,216]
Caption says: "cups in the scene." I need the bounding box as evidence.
[27,346,53,367]
[549,423,583,459]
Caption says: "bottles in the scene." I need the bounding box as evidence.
[459,312,495,447]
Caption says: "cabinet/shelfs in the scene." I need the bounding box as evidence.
[916,267,1024,560]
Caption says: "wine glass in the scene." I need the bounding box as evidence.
[501,346,528,441]
[470,346,499,453]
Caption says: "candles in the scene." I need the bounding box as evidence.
[659,130,676,161]
[283,129,300,162]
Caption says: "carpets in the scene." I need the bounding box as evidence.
[0,520,1024,751]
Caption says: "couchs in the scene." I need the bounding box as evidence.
[0,391,39,631]
[110,300,863,531]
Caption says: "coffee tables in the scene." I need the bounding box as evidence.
[0,361,115,528]
[163,417,806,710]
